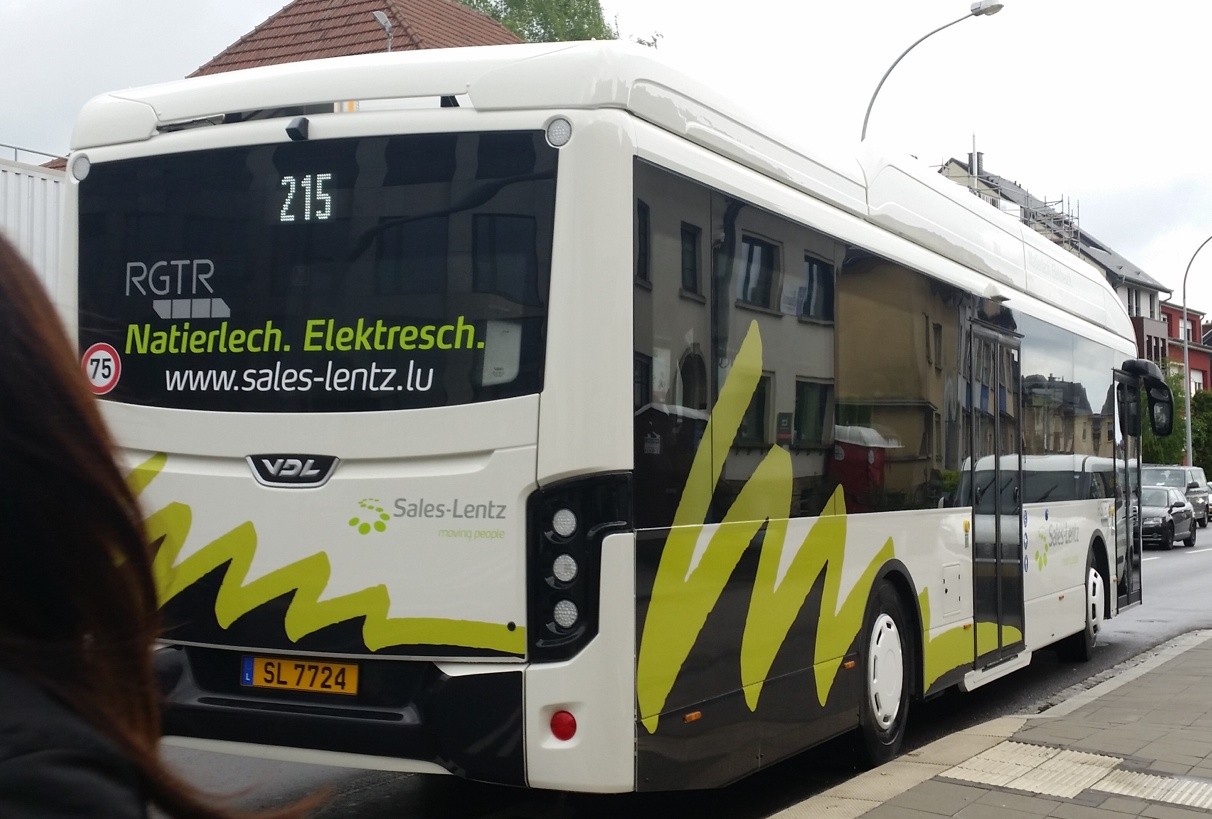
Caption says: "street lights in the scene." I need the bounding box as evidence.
[861,0,1004,143]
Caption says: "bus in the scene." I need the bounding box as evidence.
[67,33,1174,794]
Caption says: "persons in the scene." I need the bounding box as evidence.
[0,227,335,819]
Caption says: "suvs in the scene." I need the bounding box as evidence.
[1139,466,1209,527]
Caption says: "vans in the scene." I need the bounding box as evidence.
[939,454,1138,594]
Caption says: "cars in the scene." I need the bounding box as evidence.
[1140,485,1197,551]
[1207,482,1212,521]
